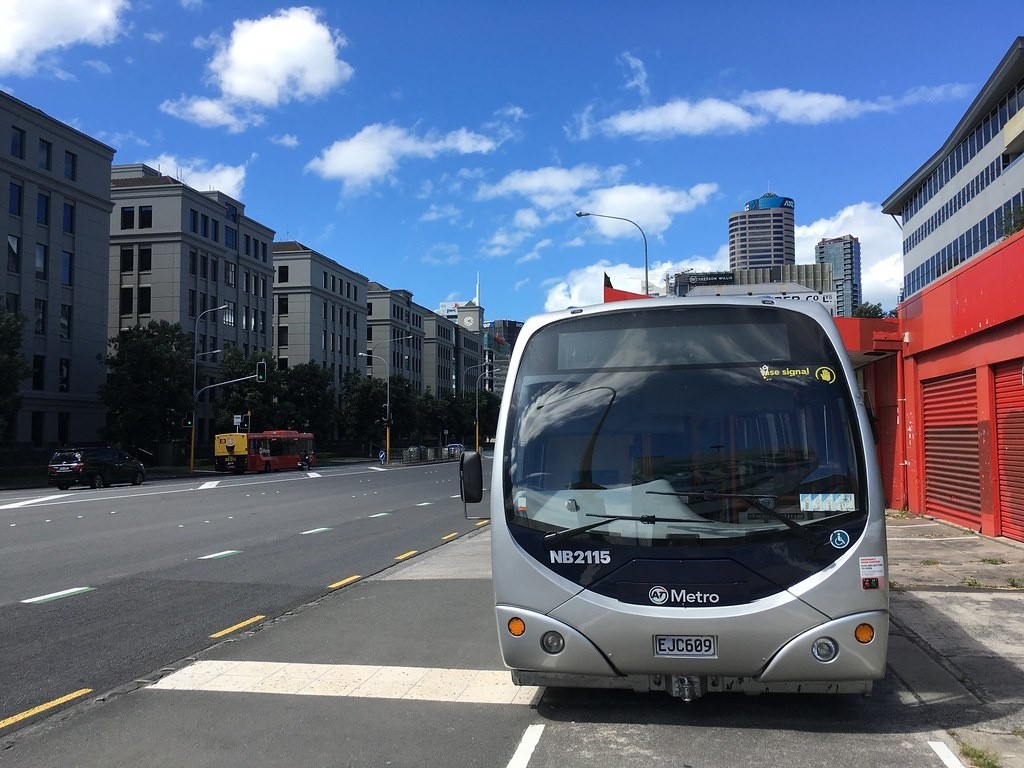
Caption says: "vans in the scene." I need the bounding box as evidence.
[48,448,146,491]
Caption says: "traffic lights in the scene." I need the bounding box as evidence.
[242,415,250,430]
[181,418,195,428]
[257,362,267,383]
[381,404,389,421]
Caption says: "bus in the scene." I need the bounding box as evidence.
[214,430,317,476]
[454,296,891,703]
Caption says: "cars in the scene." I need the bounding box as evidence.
[443,444,465,459]
[402,446,428,462]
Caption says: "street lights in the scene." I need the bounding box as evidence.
[461,360,493,455]
[474,368,500,455]
[190,349,223,472]
[368,336,414,457]
[190,304,229,471]
[576,211,650,301]
[359,352,391,466]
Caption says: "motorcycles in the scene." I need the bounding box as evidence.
[296,456,313,473]
